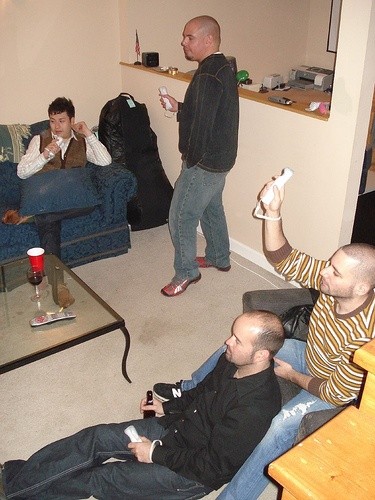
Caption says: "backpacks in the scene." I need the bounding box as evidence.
[98,89,176,233]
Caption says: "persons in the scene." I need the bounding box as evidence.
[16,97,112,310]
[159,16,239,297]
[152,168,374,499]
[1,310,283,499]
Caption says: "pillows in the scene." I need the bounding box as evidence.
[18,163,100,218]
[0,160,20,210]
[0,124,32,164]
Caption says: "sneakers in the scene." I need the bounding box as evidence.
[161,272,202,297]
[152,379,183,403]
[194,256,231,272]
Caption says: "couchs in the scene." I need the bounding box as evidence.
[27,118,139,267]
[240,285,363,455]
[0,122,42,292]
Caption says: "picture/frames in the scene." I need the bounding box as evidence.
[326,0,341,53]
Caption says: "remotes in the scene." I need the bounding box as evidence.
[30,311,76,326]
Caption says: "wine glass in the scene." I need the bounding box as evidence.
[27,267,44,302]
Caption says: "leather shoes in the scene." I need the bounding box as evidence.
[55,286,75,308]
[2,209,28,226]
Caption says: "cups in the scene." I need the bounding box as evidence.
[27,248,45,275]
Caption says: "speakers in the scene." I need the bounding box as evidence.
[142,52,159,67]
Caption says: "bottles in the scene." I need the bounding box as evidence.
[144,391,155,419]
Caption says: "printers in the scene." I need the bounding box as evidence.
[287,65,333,91]
